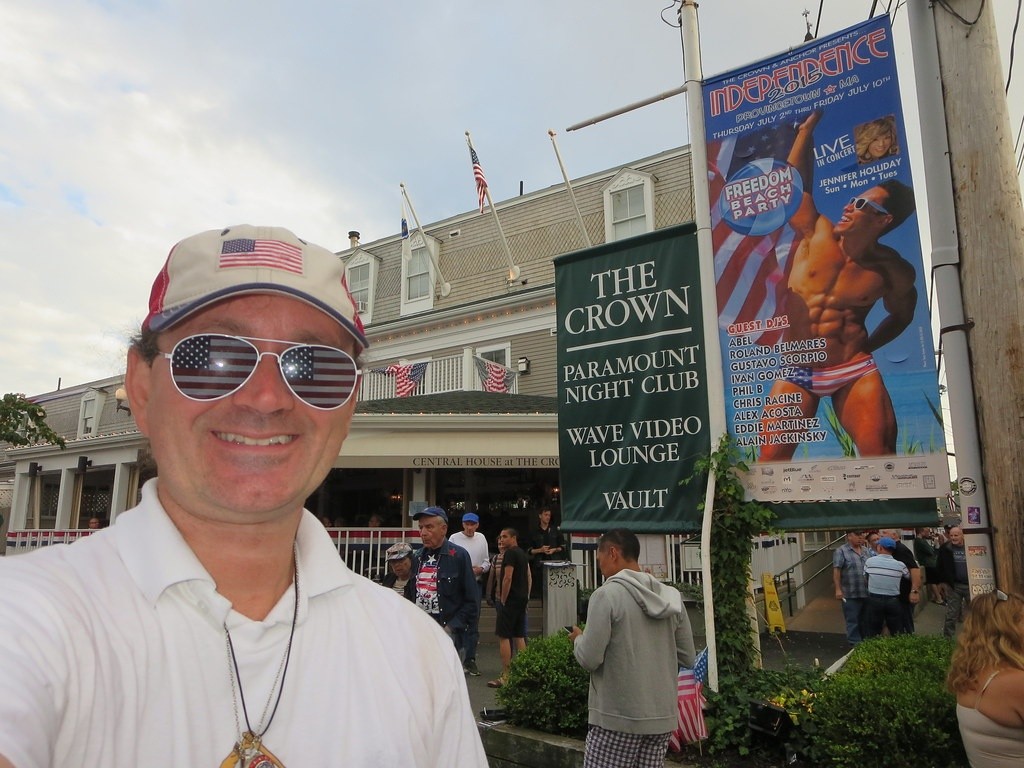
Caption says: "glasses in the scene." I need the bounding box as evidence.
[849,196,889,218]
[151,332,363,411]
[995,588,1008,609]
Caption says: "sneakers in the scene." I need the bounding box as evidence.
[463,657,479,675]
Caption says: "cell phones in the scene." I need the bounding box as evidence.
[564,626,573,634]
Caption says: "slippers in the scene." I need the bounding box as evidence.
[488,677,503,688]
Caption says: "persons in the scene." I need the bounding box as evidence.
[946,589,1024,768]
[566,528,696,768]
[448,513,490,675]
[381,506,480,650]
[486,527,532,687]
[319,515,346,527]
[757,111,918,463]
[832,527,970,649]
[526,507,567,561]
[0,224,490,768]
[89,518,100,529]
[367,512,383,527]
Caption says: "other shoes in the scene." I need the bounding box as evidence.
[933,598,949,607]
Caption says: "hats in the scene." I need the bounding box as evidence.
[462,513,478,524]
[385,542,413,564]
[846,529,865,534]
[875,538,896,549]
[141,223,370,351]
[413,506,449,524]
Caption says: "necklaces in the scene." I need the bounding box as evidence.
[416,555,441,600]
[223,542,300,768]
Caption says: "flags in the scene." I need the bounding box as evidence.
[469,146,488,214]
[401,200,412,260]
[707,116,805,348]
[373,362,427,398]
[670,647,708,751]
